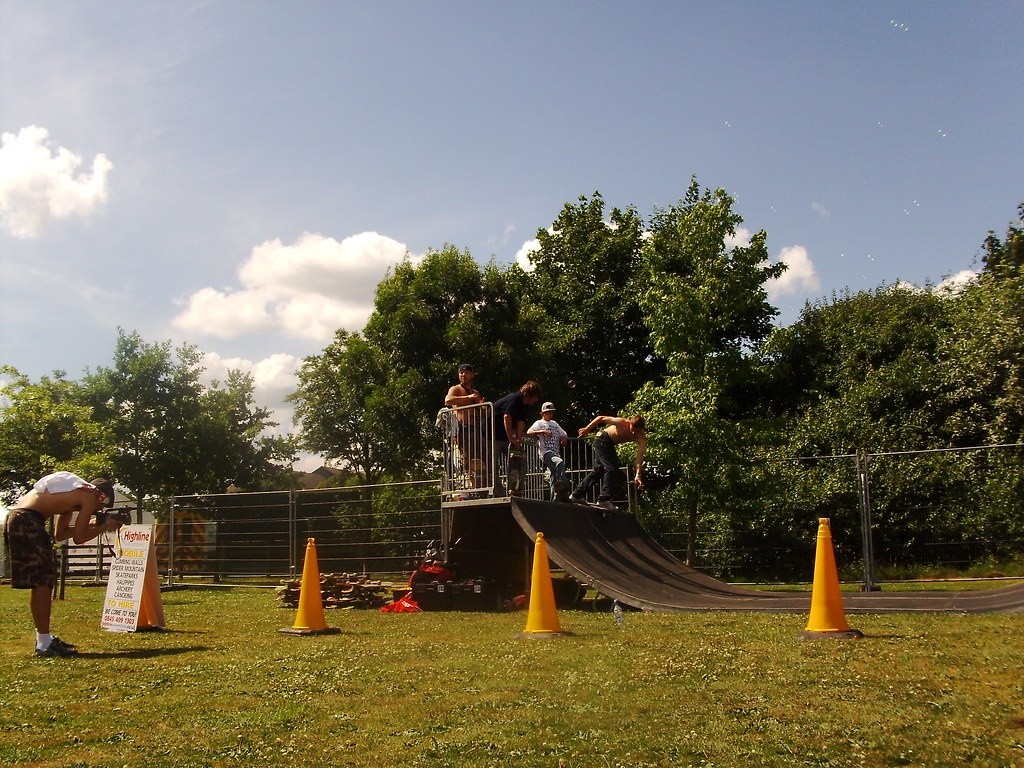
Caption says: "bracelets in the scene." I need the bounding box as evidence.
[104,522,108,528]
[636,473,641,477]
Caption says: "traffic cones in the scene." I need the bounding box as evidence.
[278,539,342,634]
[799,518,865,638]
[515,532,577,638]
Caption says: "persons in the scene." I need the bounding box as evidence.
[527,402,574,504]
[4,472,123,657]
[568,414,646,510]
[483,380,542,498]
[444,364,491,498]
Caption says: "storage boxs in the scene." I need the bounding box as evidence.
[392,590,409,602]
[411,582,483,612]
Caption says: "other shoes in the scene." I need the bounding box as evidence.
[569,493,587,506]
[595,500,618,511]
[35,636,78,657]
[507,490,524,498]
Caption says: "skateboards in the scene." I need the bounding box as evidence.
[467,458,498,498]
[578,500,619,520]
[554,479,569,503]
[506,443,525,497]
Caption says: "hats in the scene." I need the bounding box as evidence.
[458,363,473,373]
[90,478,114,508]
[541,402,556,412]
